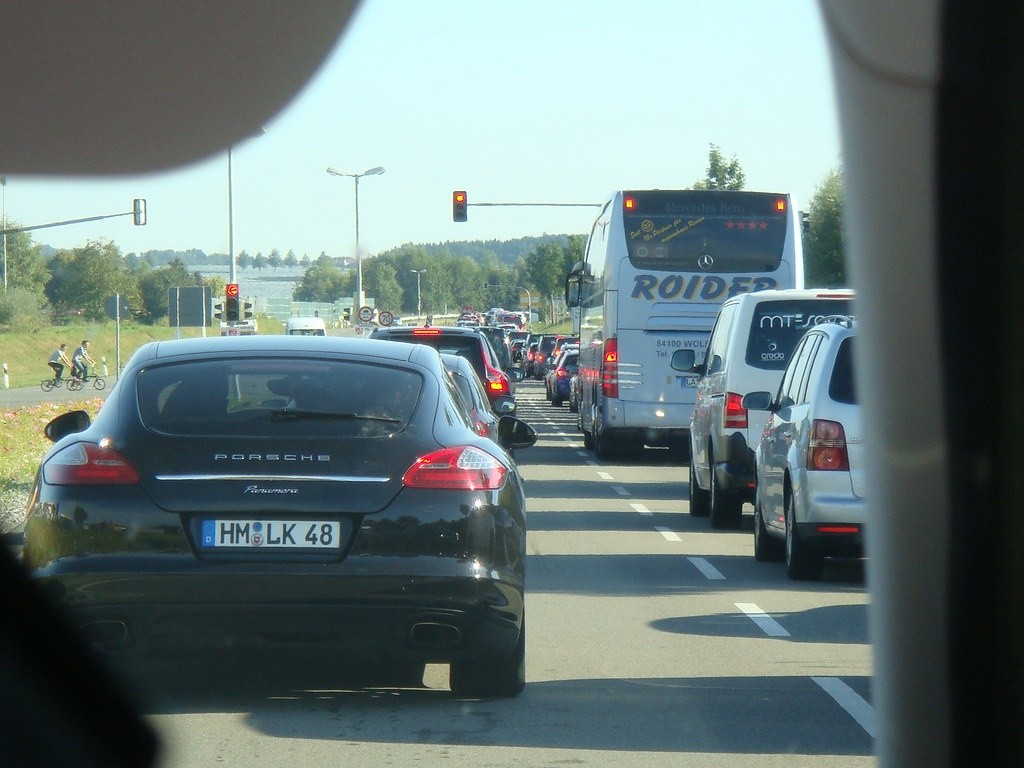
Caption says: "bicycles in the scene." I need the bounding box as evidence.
[66,362,105,391]
[40,366,83,392]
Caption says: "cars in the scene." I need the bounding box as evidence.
[580,331,603,379]
[506,330,579,413]
[440,354,500,444]
[458,308,530,336]
[581,315,603,337]
[556,297,563,301]
[22,335,539,701]
[285,317,326,336]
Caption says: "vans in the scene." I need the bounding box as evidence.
[671,288,858,529]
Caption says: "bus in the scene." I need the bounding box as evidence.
[566,190,805,463]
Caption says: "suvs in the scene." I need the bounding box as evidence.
[368,327,518,422]
[741,315,865,582]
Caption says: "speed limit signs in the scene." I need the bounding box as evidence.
[379,312,393,325]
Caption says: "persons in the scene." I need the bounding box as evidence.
[47,343,71,382]
[72,340,96,382]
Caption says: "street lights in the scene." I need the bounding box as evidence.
[411,269,427,317]
[325,166,387,325]
[227,125,267,336]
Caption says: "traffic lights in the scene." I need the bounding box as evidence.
[244,302,252,319]
[484,282,488,289]
[214,304,222,319]
[344,308,352,321]
[226,284,240,321]
[453,191,468,223]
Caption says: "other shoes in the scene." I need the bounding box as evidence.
[59,377,64,380]
[83,379,89,382]
